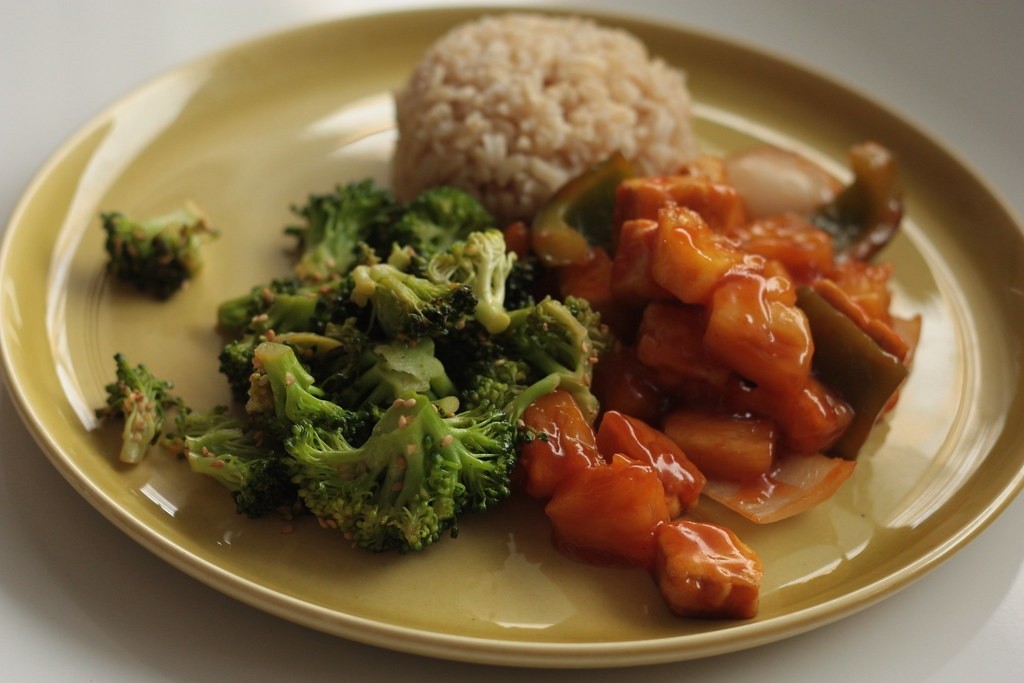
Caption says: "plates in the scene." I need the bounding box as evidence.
[0,0,1024,668]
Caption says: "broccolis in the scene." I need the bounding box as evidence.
[103,188,608,550]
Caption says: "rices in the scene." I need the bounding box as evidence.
[393,13,691,220]
